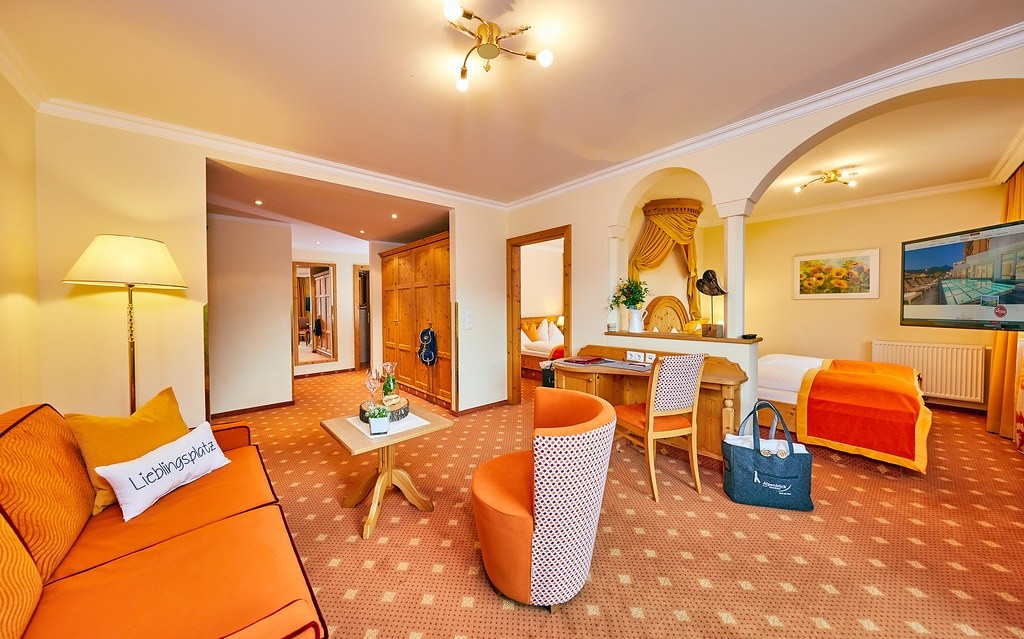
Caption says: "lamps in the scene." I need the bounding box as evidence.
[557,316,565,331]
[440,0,554,93]
[63,233,190,418]
[794,169,858,192]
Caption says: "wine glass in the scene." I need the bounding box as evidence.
[365,369,380,409]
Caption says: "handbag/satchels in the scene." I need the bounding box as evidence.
[722,399,814,513]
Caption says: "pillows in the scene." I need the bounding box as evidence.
[522,320,564,352]
[96,422,232,523]
[65,387,191,516]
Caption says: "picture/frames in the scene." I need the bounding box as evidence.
[794,249,879,300]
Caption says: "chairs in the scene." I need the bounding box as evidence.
[612,354,703,502]
[472,386,617,614]
[904,277,938,304]
[298,317,311,346]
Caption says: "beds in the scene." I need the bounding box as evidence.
[521,316,566,382]
[754,354,934,475]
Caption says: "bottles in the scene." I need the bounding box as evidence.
[382,362,399,399]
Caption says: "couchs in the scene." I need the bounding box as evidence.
[1,404,329,639]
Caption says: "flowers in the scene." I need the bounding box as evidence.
[365,405,388,418]
[610,276,649,310]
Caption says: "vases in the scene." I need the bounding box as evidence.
[628,308,647,333]
[368,418,388,434]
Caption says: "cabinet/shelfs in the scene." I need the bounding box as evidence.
[311,269,333,358]
[378,229,453,409]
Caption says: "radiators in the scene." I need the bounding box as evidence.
[872,340,986,404]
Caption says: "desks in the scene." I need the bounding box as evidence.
[555,344,748,461]
[320,404,455,540]
